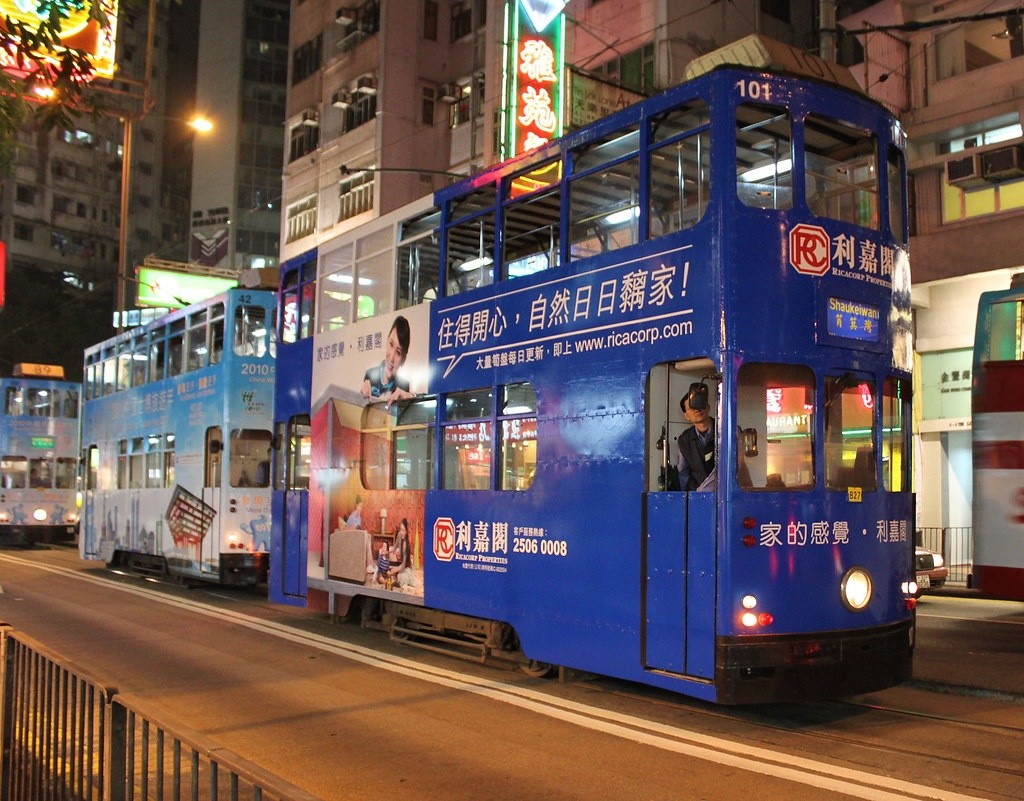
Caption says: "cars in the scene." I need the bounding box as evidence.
[915,545,949,600]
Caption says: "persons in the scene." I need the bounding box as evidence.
[360,315,428,409]
[30,468,43,488]
[255,447,271,488]
[346,494,422,589]
[676,391,753,492]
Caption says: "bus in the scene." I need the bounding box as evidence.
[269,64,922,715]
[81,283,280,586]
[0,363,79,546]
